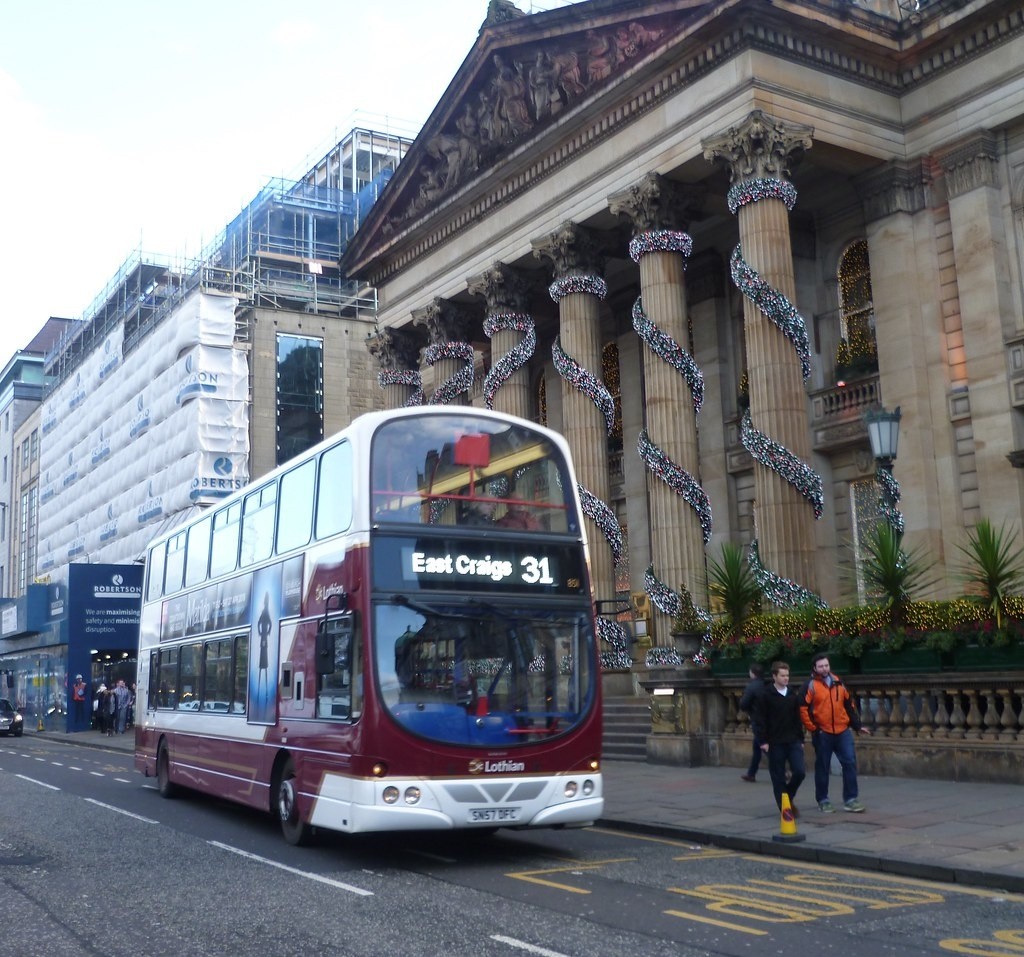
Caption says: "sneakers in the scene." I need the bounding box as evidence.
[844,800,865,812]
[818,803,836,813]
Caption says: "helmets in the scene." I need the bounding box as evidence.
[76,674,82,679]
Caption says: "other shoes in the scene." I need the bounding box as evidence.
[741,774,756,783]
[114,729,119,736]
[101,729,106,734]
[785,769,792,779]
[107,730,113,737]
[120,731,126,736]
[126,724,130,730]
[791,803,800,818]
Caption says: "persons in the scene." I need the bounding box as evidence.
[455,636,501,714]
[463,492,543,531]
[418,19,664,199]
[798,655,870,812]
[396,638,424,696]
[92,681,136,736]
[72,675,88,722]
[739,665,792,782]
[756,664,807,817]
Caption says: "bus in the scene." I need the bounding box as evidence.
[135,406,642,848]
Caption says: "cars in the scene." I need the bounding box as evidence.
[0,698,23,737]
